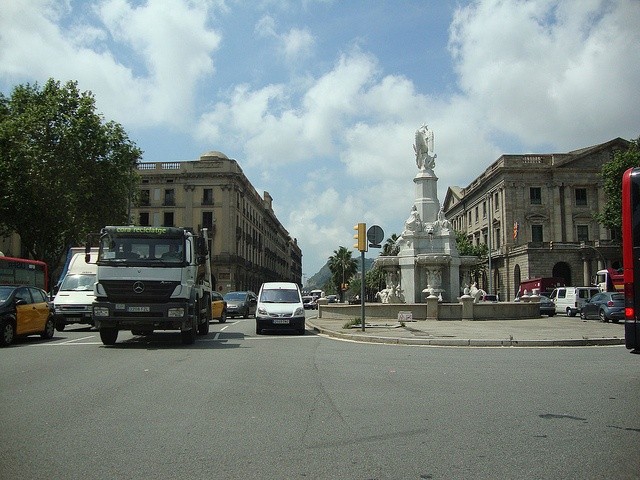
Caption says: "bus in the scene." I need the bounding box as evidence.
[623,169,640,352]
[308,289,325,300]
[0,252,47,292]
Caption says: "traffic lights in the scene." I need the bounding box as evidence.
[550,240,554,252]
[514,222,519,240]
[353,222,366,251]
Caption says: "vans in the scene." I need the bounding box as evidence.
[52,245,123,332]
[250,282,310,335]
[550,287,600,317]
[302,296,315,305]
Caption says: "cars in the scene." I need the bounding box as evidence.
[478,294,497,303]
[580,292,625,323]
[211,291,227,324]
[0,283,56,348]
[327,294,336,303]
[303,299,319,311]
[223,290,257,319]
[538,296,556,318]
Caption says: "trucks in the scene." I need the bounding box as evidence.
[591,268,624,294]
[514,276,567,303]
[84,225,213,346]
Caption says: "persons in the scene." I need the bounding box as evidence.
[409,204,423,232]
[439,203,449,228]
[160,244,181,263]
[523,288,528,295]
[531,288,537,295]
[118,243,141,260]
